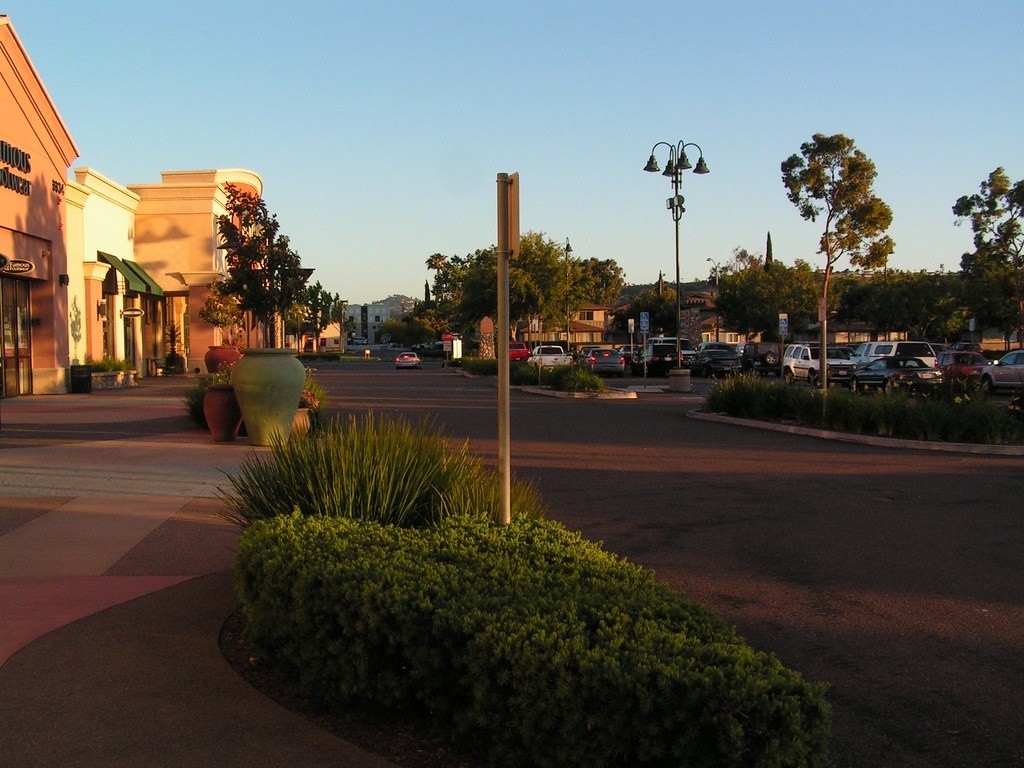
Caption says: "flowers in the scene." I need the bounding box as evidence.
[299,389,320,414]
[204,357,235,386]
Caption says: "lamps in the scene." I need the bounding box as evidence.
[60,274,69,286]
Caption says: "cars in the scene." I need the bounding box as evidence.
[577,343,741,379]
[783,340,1024,396]
[394,352,425,370]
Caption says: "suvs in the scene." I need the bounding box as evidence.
[737,342,781,376]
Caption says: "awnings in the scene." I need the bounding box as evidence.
[98,251,147,292]
[122,258,164,296]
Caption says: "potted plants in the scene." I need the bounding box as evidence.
[218,179,305,446]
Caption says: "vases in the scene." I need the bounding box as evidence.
[204,346,241,374]
[204,384,243,442]
[290,409,311,442]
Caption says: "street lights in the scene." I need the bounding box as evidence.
[216,203,275,349]
[642,141,711,369]
[565,237,573,352]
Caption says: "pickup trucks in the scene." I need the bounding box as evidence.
[527,345,573,369]
[509,341,532,361]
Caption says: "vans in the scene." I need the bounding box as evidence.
[647,337,698,368]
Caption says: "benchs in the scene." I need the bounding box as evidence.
[153,359,175,377]
